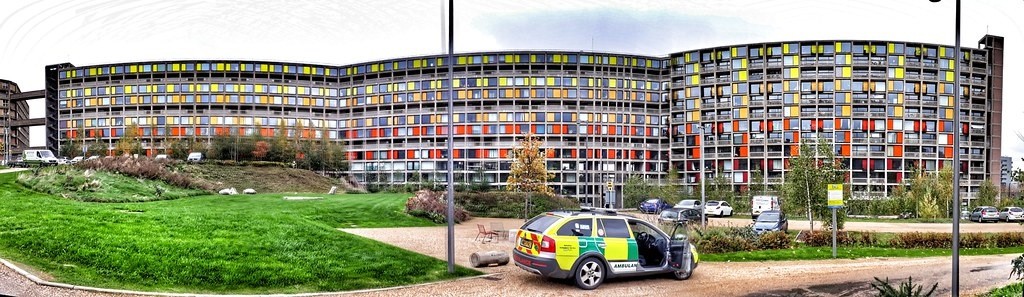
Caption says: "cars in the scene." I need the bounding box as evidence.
[705,200,733,217]
[8,156,23,168]
[657,209,709,227]
[1000,206,1024,223]
[673,200,701,209]
[752,210,788,234]
[969,205,1000,223]
[639,199,672,213]
[188,152,203,161]
[59,156,100,164]
[156,154,171,160]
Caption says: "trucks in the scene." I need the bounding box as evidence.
[752,196,781,219]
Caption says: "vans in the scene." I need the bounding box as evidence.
[22,149,58,168]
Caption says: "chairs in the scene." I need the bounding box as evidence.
[489,221,509,239]
[475,224,498,244]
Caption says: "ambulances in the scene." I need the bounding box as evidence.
[512,206,699,290]
